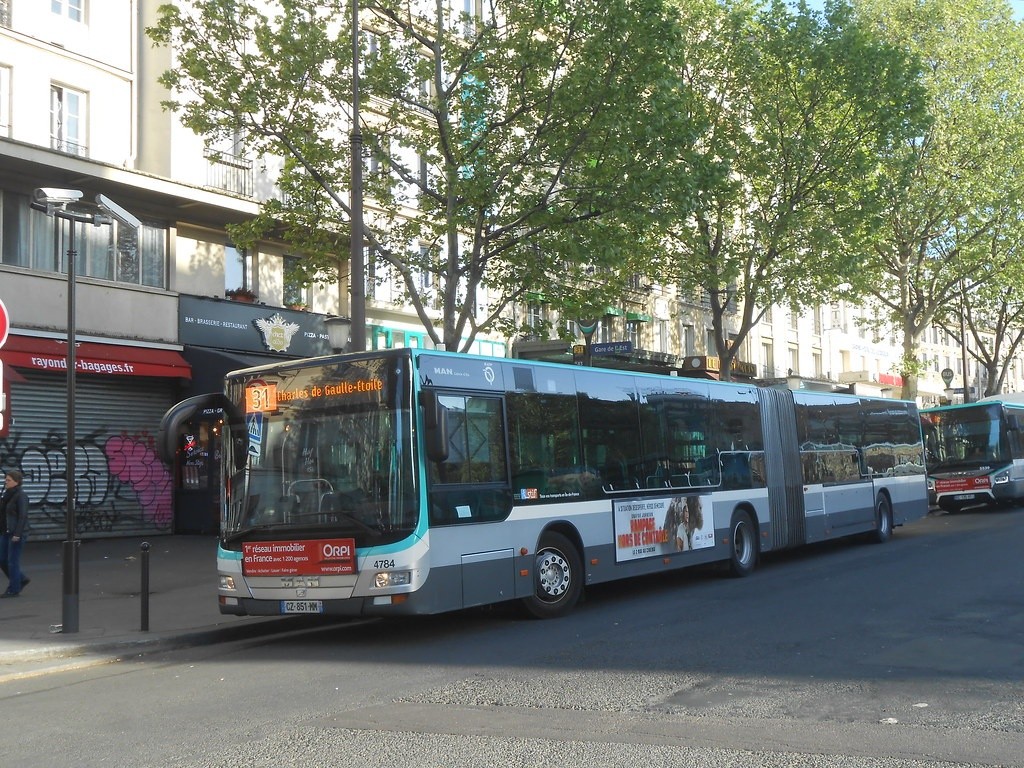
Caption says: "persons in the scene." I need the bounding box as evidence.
[677,506,690,551]
[0,472,31,598]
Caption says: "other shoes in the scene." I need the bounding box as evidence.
[0,587,18,598]
[19,577,31,593]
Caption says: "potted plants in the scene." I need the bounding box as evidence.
[285,301,306,310]
[226,288,257,303]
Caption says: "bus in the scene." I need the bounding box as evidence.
[159,347,934,618]
[918,394,1023,512]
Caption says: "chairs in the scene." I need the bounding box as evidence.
[603,452,717,495]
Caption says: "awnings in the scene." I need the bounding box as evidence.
[0,336,192,380]
[184,345,289,397]
